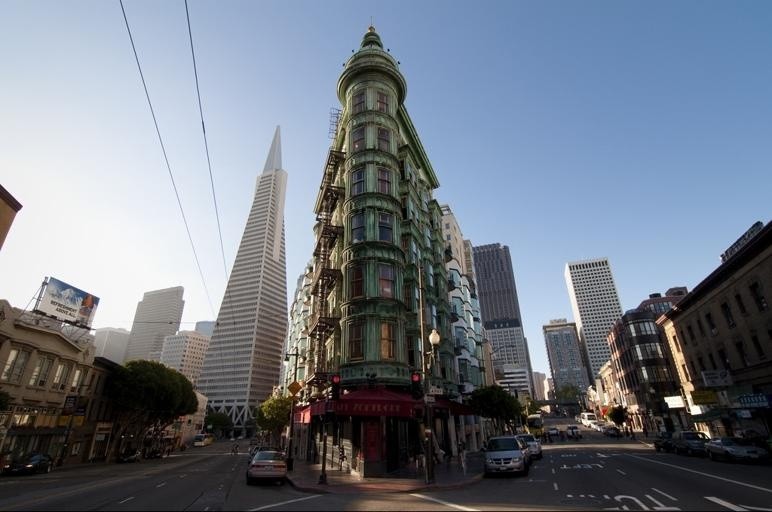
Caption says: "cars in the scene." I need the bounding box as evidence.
[517,433,543,460]
[480,435,531,476]
[3,452,54,477]
[548,425,582,439]
[673,430,767,464]
[590,421,620,437]
[246,450,289,486]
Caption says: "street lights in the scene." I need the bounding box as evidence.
[284,346,300,472]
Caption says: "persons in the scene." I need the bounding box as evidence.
[614,423,649,441]
[535,427,579,443]
[231,440,241,455]
[82,295,94,309]
[458,441,471,476]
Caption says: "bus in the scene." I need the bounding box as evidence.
[526,413,544,437]
[194,433,216,447]
[581,412,598,428]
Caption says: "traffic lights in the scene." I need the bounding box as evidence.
[331,373,341,401]
[411,372,424,400]
